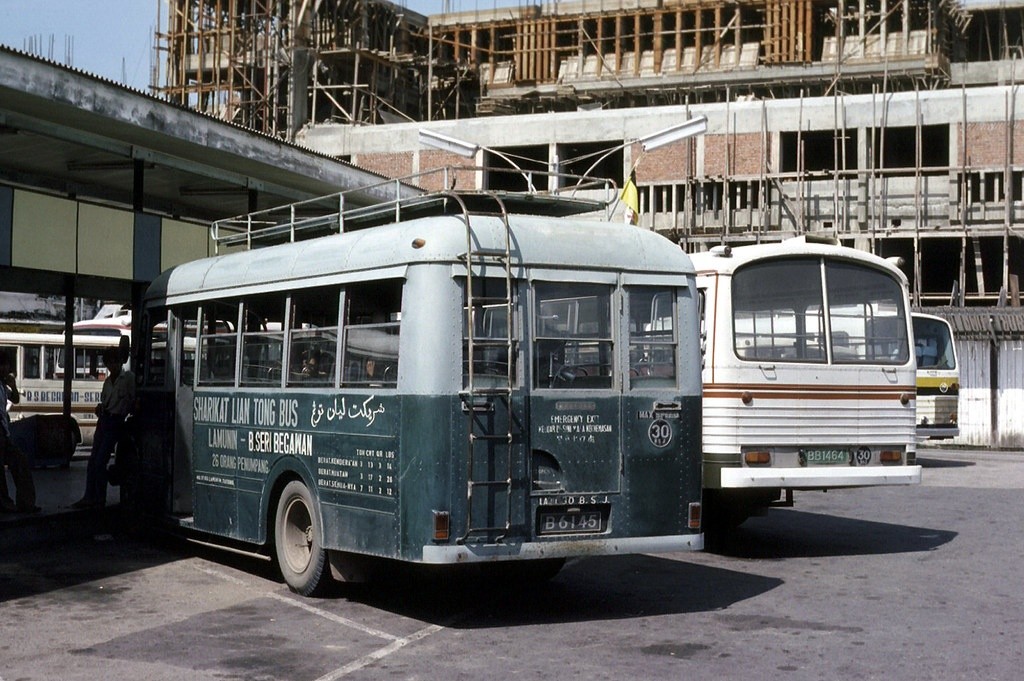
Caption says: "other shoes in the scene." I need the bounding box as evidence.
[0,500,17,513]
[21,504,41,514]
[68,497,102,510]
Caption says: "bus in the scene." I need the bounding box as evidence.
[0,329,202,470]
[685,236,928,530]
[645,304,961,446]
[127,166,706,597]
[52,311,235,386]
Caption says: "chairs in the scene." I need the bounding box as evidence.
[203,334,643,393]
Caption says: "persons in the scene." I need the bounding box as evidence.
[71,346,137,511]
[209,346,391,389]
[0,353,45,516]
